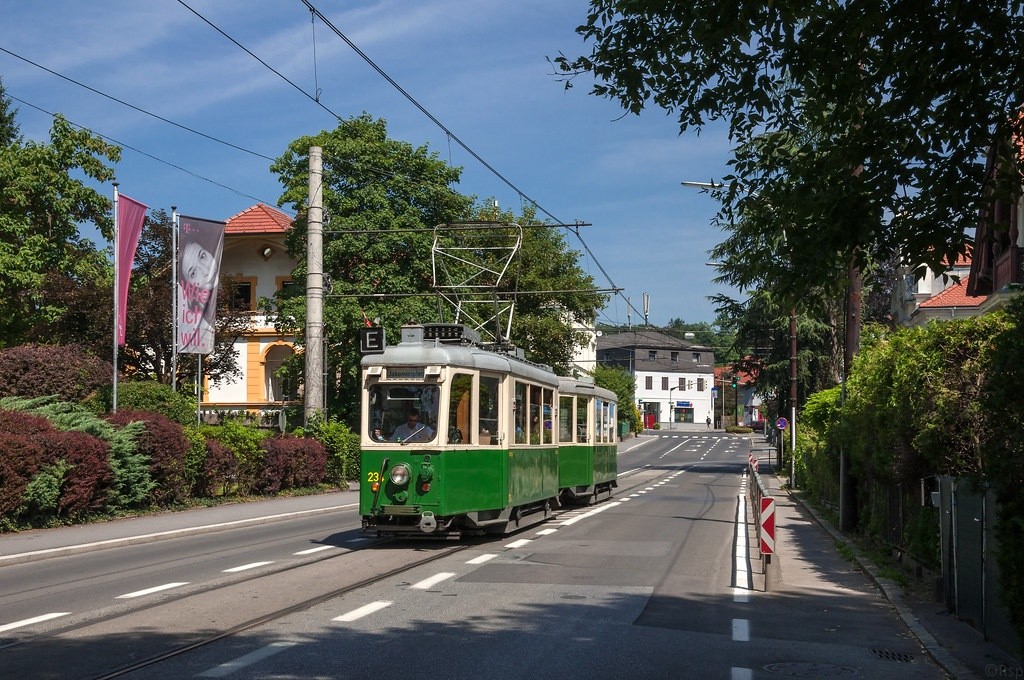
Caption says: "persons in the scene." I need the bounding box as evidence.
[479,421,490,436]
[390,407,433,442]
[706,416,711,430]
[177,235,218,314]
[374,427,385,440]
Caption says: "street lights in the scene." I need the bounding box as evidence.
[680,180,796,489]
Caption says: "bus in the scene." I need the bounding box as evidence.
[356,319,620,541]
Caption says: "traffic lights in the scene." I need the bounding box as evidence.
[733,376,740,389]
[688,379,694,390]
[703,379,707,389]
